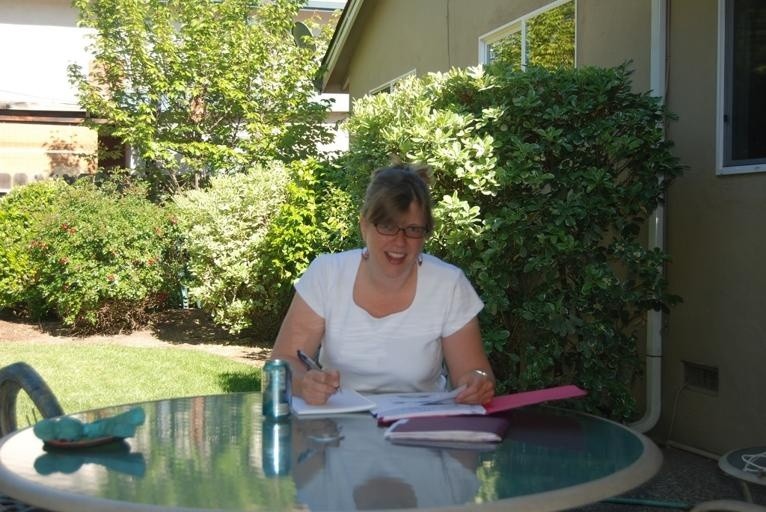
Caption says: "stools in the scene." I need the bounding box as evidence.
[719,441,765,508]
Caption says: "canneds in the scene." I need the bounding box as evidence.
[260,359,292,420]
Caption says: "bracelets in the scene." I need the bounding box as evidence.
[473,368,490,376]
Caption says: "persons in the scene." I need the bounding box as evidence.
[270,162,498,407]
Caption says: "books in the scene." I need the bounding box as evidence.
[383,413,507,441]
[291,387,376,417]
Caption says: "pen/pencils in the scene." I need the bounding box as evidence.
[296,350,343,394]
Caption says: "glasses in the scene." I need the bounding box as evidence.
[369,220,428,239]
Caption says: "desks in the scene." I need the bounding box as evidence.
[0,383,665,510]
